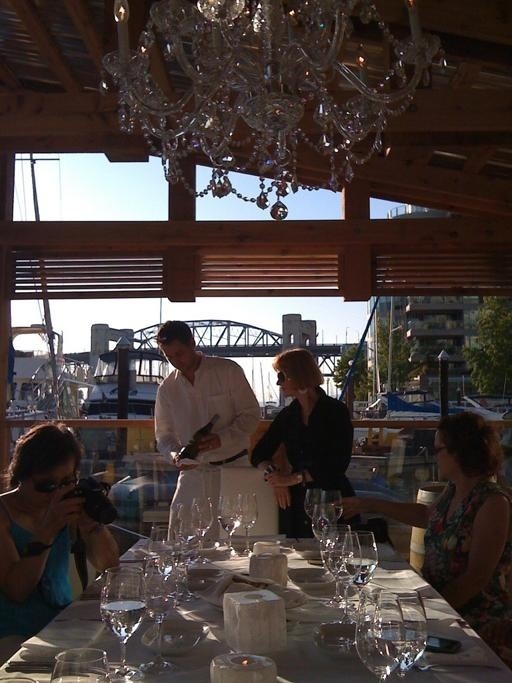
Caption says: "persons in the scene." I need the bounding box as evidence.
[0,421,121,664]
[154,321,279,539]
[421,410,511,670]
[249,348,365,537]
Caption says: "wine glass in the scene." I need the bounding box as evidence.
[303,483,430,683]
[99,492,260,682]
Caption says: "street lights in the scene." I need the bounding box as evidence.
[333,370,340,400]
[348,360,354,414]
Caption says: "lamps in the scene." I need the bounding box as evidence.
[93,0,456,226]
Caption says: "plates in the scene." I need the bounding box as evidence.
[287,567,335,587]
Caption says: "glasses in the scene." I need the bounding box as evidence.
[429,446,448,457]
[276,371,286,382]
[33,473,76,494]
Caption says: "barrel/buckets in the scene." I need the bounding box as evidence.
[409,480,449,577]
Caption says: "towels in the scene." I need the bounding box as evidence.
[14,615,108,658]
[373,559,434,596]
[421,620,487,664]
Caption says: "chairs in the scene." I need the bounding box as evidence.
[215,466,281,536]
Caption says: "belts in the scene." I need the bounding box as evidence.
[208,449,248,467]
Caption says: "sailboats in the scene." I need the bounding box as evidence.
[250,360,283,419]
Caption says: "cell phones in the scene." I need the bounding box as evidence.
[425,634,461,653]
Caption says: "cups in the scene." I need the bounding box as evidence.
[1,676,39,683]
[49,647,111,683]
[252,536,281,553]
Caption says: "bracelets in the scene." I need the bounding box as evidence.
[296,468,307,488]
[263,463,279,481]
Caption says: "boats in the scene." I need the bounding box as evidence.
[73,335,170,459]
[353,372,512,420]
[10,322,94,457]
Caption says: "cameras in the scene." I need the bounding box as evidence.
[62,482,117,524]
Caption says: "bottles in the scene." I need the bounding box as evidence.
[178,410,220,461]
